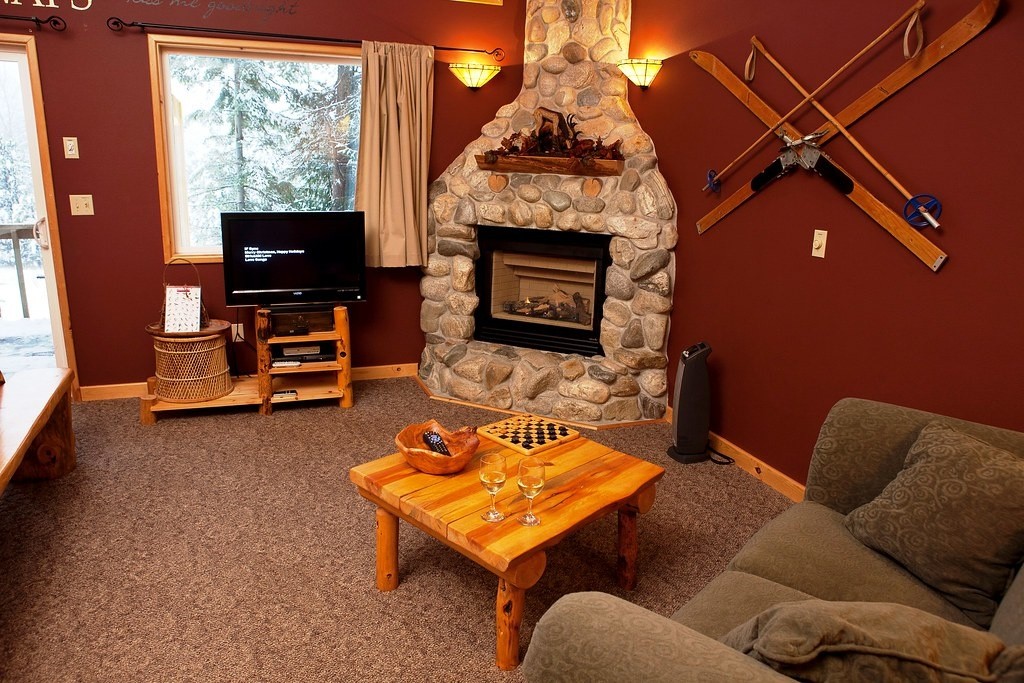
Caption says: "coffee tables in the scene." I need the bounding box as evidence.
[349,413,667,670]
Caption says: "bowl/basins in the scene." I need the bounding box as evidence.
[394,419,480,474]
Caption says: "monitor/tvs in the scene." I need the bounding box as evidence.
[221,210,367,308]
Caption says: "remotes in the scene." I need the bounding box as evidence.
[423,431,452,458]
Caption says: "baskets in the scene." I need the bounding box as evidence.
[158,258,210,328]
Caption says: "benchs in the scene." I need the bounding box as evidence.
[0,369,76,497]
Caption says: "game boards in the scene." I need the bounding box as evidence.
[476,413,580,456]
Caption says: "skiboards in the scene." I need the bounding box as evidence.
[688,0,1006,273]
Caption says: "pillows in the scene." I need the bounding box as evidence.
[715,601,1024,683]
[844,420,1024,628]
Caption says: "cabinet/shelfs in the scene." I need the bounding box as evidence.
[139,306,353,425]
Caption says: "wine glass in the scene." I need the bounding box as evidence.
[518,457,546,526]
[479,453,507,522]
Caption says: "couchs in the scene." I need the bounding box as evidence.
[526,397,1024,683]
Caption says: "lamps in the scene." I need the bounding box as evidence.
[617,59,663,89]
[448,64,502,90]
